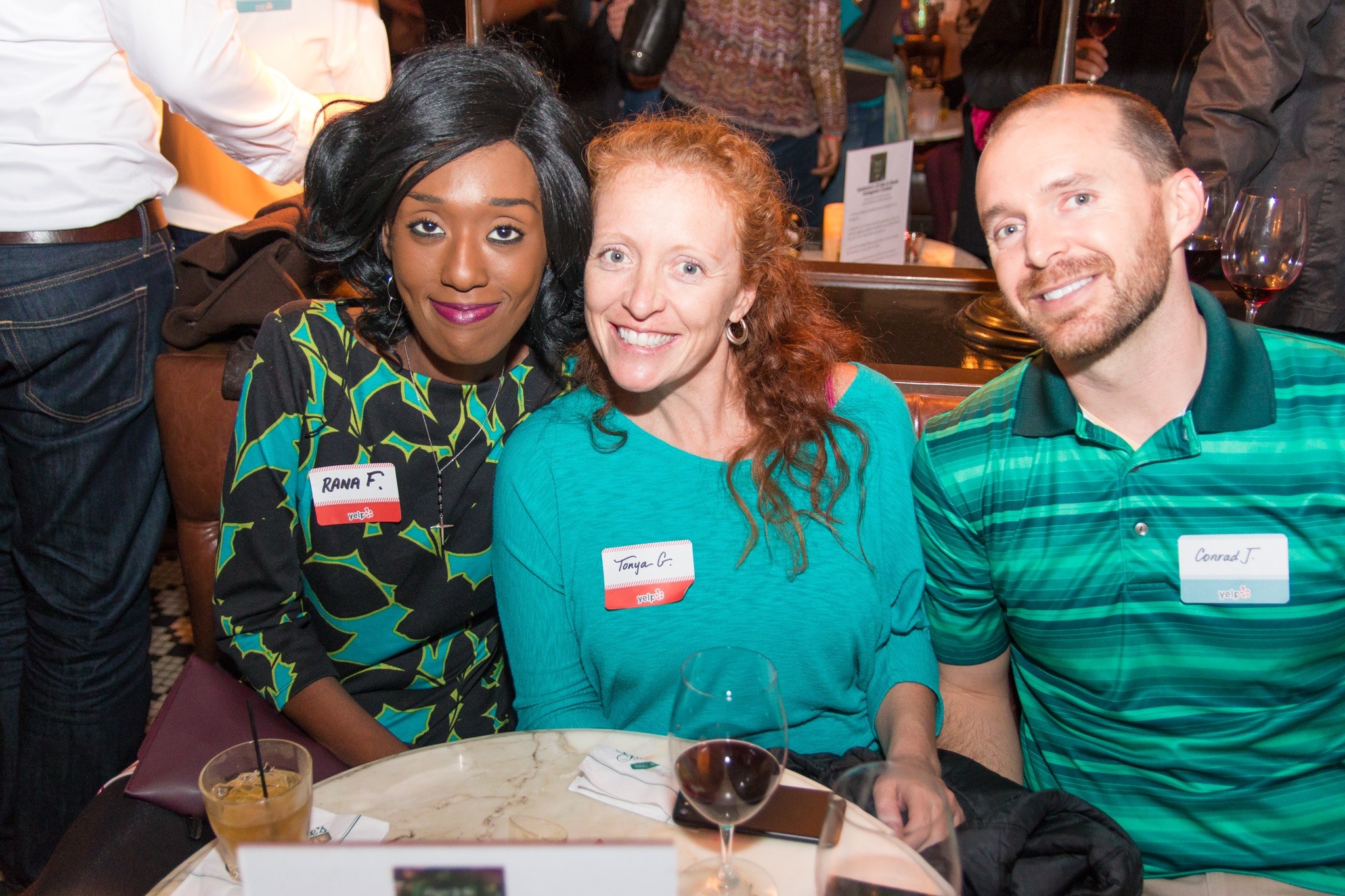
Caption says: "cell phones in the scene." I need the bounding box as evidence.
[673,782,846,846]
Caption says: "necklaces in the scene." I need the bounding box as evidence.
[404,335,505,545]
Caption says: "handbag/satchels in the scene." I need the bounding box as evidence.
[617,0,688,92]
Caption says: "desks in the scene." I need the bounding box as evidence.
[909,107,966,239]
[143,728,960,896]
[793,225,989,270]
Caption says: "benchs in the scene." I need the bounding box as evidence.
[148,258,1004,669]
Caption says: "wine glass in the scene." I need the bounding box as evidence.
[1221,187,1307,324]
[1085,0,1123,85]
[670,647,787,896]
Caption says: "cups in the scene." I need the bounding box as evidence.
[815,761,963,895]
[1178,171,1235,283]
[912,88,943,129]
[199,739,313,881]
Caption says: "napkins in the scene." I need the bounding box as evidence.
[570,742,700,835]
[171,807,390,896]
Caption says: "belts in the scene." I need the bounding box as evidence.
[0,198,170,246]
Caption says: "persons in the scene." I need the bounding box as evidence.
[0,0,351,896]
[604,0,849,212]
[1178,0,1345,324]
[910,87,1345,896]
[491,110,964,853]
[951,0,1210,269]
[27,45,593,895]
[481,0,635,107]
